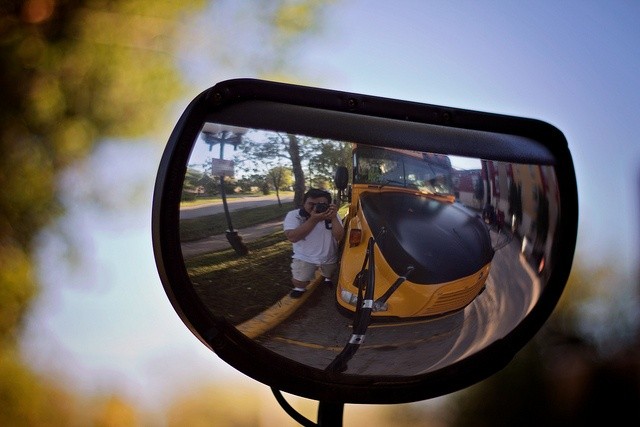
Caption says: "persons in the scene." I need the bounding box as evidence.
[283,187,345,298]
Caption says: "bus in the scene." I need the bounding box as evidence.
[335,142,519,325]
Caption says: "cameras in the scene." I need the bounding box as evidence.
[313,201,336,217]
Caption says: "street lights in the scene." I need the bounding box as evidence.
[202,122,247,260]
